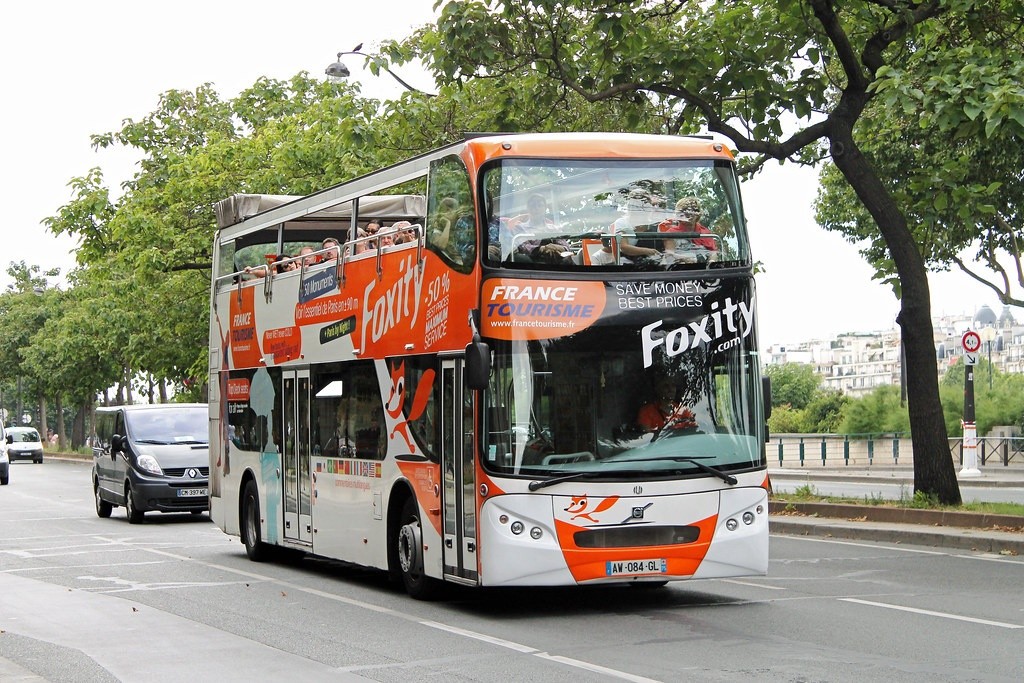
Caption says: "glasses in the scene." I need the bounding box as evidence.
[281,261,293,269]
[366,229,377,234]
[402,230,413,234]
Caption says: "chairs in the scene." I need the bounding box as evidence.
[495,211,678,265]
[597,386,633,458]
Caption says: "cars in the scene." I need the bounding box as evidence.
[6,426,44,464]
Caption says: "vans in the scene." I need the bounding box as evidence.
[92,401,212,524]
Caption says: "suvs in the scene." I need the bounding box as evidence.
[0,419,14,486]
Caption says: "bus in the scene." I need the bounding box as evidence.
[211,132,772,590]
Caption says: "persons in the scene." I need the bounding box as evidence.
[428,194,575,265]
[139,416,170,438]
[51,434,59,442]
[256,370,474,461]
[593,188,718,265]
[173,418,186,434]
[637,371,698,432]
[245,219,415,276]
